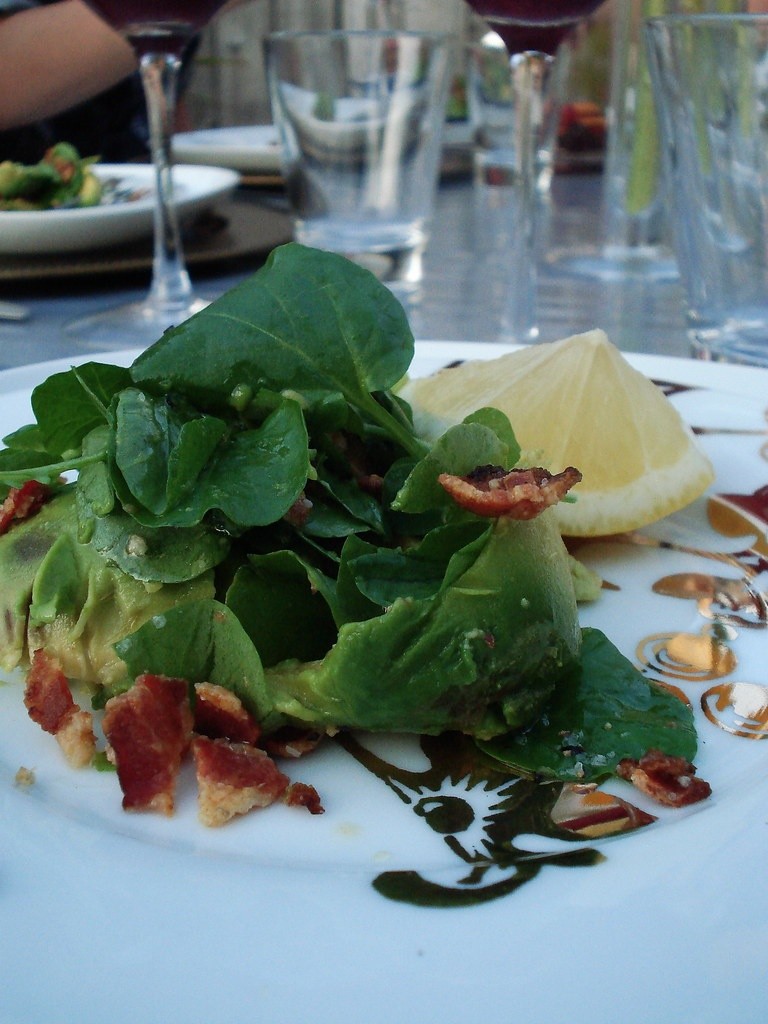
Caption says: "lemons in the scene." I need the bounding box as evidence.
[394,329,716,537]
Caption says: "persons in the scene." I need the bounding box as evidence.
[0,0,225,166]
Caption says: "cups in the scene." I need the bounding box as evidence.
[642,13,768,368]
[264,1,462,300]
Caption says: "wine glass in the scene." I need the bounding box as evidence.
[464,1,605,345]
[60,0,265,354]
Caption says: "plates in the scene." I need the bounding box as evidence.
[173,125,280,174]
[0,342,768,1024]
[0,163,242,256]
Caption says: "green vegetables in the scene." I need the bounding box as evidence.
[0,141,102,210]
[0,240,703,781]
